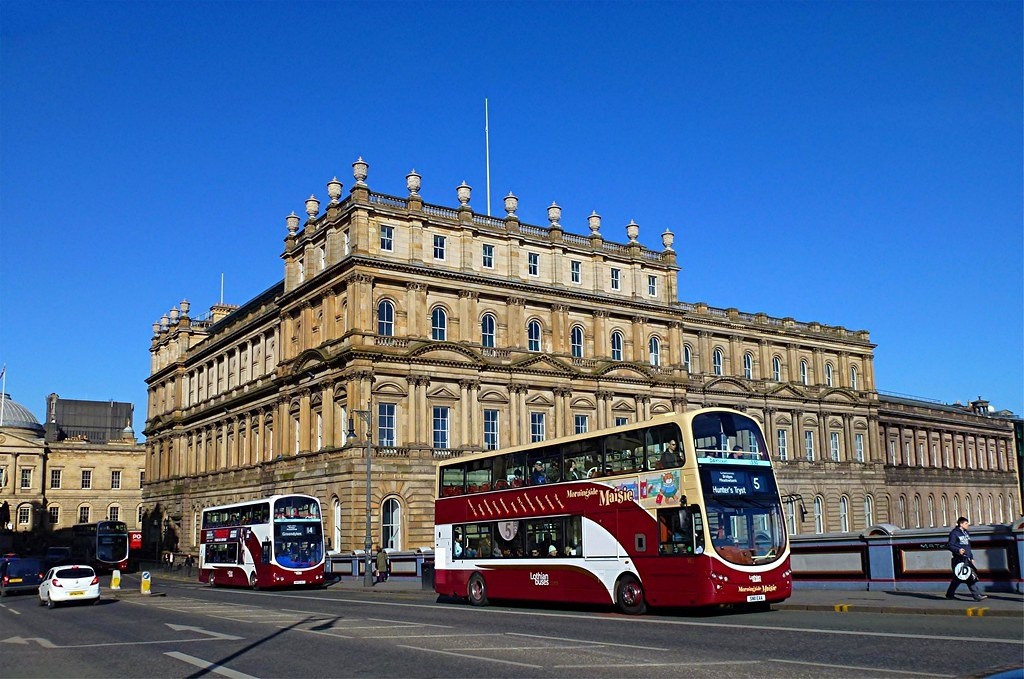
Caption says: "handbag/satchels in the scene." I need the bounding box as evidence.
[951,553,980,584]
[376,570,380,576]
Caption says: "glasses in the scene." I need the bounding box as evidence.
[531,552,537,554]
[669,444,676,445]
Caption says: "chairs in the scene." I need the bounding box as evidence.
[442,460,663,498]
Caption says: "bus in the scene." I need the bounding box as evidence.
[199,493,332,591]
[72,520,130,573]
[433,403,807,615]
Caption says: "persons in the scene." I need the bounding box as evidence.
[945,516,988,602]
[275,511,286,519]
[516,549,523,557]
[230,514,238,526]
[531,548,540,558]
[530,461,561,486]
[503,548,511,557]
[376,548,387,582]
[240,512,259,525]
[184,553,195,577]
[163,552,174,571]
[209,545,215,563]
[659,440,685,469]
[728,445,743,459]
[706,446,717,458]
[566,459,581,481]
[546,545,558,558]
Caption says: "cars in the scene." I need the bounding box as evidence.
[38,565,101,609]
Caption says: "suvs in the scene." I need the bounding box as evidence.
[0,553,47,598]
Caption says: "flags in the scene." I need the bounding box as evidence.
[0,366,5,378]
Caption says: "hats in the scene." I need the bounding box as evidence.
[536,460,542,466]
[549,545,556,552]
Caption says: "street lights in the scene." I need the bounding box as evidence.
[346,408,373,588]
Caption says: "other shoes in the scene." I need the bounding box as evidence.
[946,595,962,600]
[975,595,988,602]
